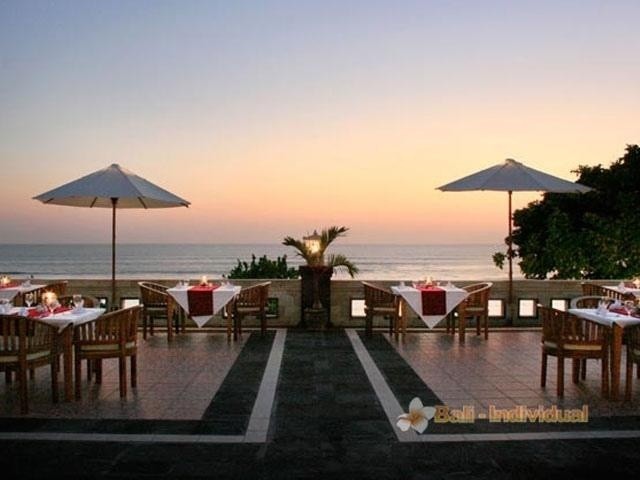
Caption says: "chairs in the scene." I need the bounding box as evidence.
[357,281,639,412]
[0,273,273,413]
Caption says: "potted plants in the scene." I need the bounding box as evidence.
[281,223,360,330]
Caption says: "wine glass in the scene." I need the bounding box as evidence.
[25,294,33,310]
[47,300,58,319]
[73,294,83,312]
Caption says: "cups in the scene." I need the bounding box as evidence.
[619,281,625,291]
[425,275,433,285]
[0,299,10,315]
[624,301,635,320]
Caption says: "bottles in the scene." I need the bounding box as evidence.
[598,296,607,315]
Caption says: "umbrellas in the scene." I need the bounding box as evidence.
[433,156,598,329]
[32,161,194,313]
